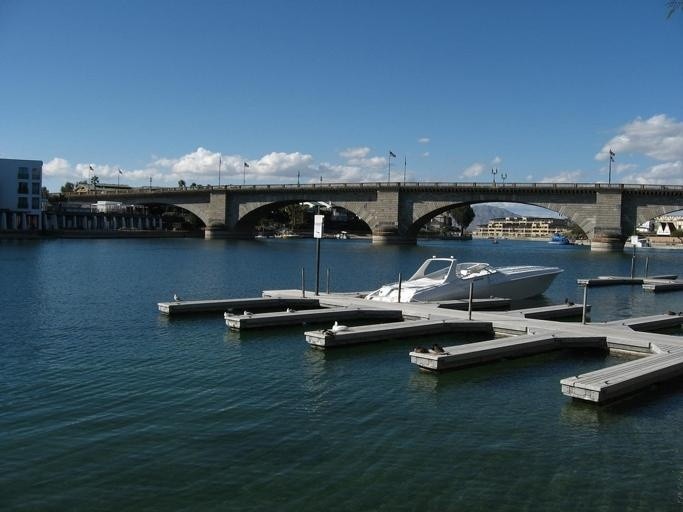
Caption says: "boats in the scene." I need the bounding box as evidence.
[491,238,498,243]
[256,226,354,242]
[549,233,568,246]
[367,255,567,305]
